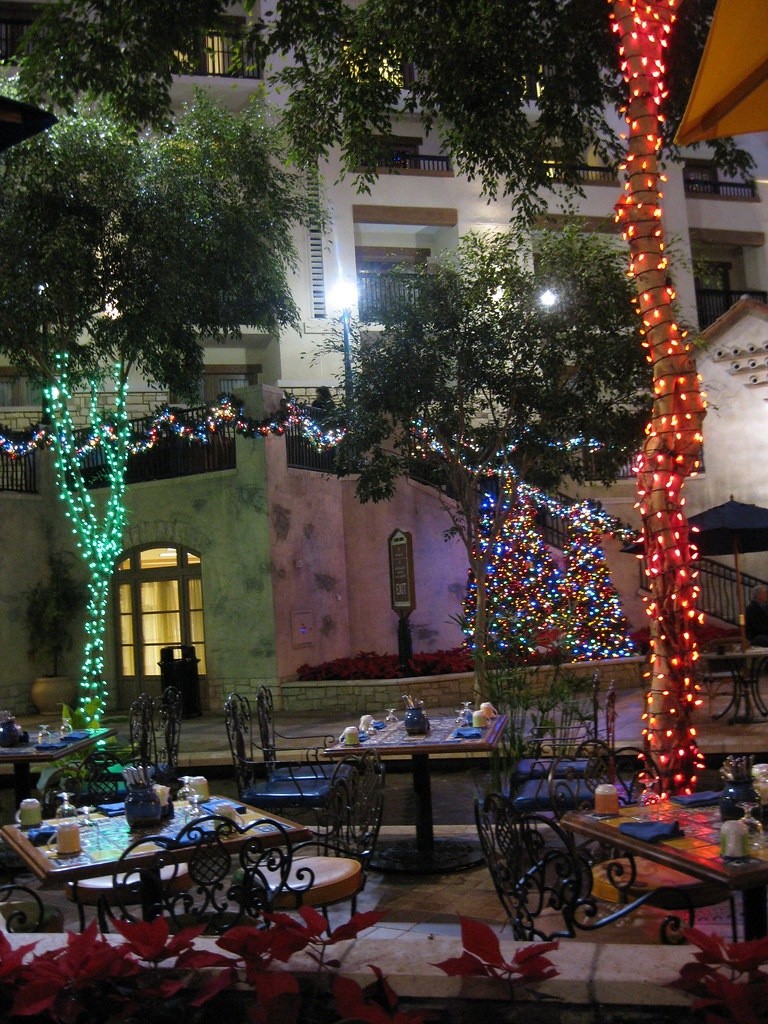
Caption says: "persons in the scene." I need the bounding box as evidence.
[311,386,337,411]
[744,585,768,648]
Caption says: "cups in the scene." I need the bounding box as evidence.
[480,702,498,719]
[721,820,750,859]
[338,726,359,745]
[47,822,82,857]
[189,776,209,802]
[594,784,619,816]
[359,715,374,734]
[472,710,491,727]
[214,802,245,832]
[15,798,42,828]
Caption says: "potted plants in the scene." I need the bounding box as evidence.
[23,544,91,715]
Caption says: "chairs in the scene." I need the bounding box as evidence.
[699,638,756,716]
[0,686,386,935]
[475,739,768,945]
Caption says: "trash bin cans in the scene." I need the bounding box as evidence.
[157,646,202,719]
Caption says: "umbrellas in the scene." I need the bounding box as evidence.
[619,494,768,650]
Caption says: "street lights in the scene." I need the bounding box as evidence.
[333,279,359,416]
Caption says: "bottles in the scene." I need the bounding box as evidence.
[720,774,761,822]
[404,707,427,736]
[125,779,161,829]
[0,719,19,748]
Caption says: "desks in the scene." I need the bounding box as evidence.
[562,791,768,943]
[321,715,508,875]
[0,729,119,812]
[0,795,310,925]
[698,646,768,724]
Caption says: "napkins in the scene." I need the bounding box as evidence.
[199,798,246,816]
[373,722,385,730]
[455,727,481,739]
[155,825,216,850]
[618,821,685,843]
[671,791,722,808]
[28,825,57,846]
[61,733,90,740]
[358,734,369,741]
[98,802,126,816]
[34,743,68,750]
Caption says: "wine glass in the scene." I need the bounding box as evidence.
[176,775,198,815]
[635,778,661,822]
[76,805,99,852]
[460,701,473,724]
[55,792,79,825]
[60,717,73,738]
[454,710,469,730]
[38,724,51,745]
[385,708,399,731]
[736,802,764,850]
